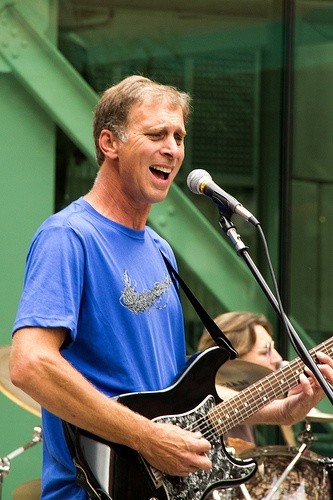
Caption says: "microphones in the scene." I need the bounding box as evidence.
[187,168,261,228]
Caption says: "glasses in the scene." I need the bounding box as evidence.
[265,343,272,353]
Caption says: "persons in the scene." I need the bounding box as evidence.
[193,311,283,458]
[8,75,333,500]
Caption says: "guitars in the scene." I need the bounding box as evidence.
[74,335,333,500]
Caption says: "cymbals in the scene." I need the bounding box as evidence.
[305,407,333,423]
[0,344,41,419]
[185,355,289,401]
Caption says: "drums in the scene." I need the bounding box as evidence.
[214,445,333,499]
